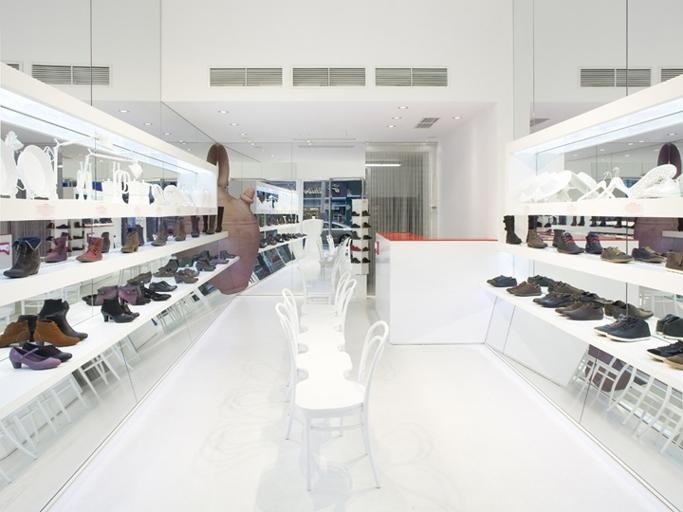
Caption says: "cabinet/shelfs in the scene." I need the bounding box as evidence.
[302,177,366,243]
[484,73,683,512]
[0,62,240,511]
[228,179,305,255]
[350,198,372,275]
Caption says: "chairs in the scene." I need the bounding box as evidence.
[276,237,389,493]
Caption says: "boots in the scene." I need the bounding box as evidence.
[15,315,37,343]
[570,216,576,226]
[121,218,128,246]
[260,214,285,227]
[101,232,110,252]
[77,237,103,262]
[37,299,87,340]
[502,216,521,244]
[526,216,543,242]
[190,216,200,237]
[297,216,299,223]
[201,215,208,232]
[151,223,168,245]
[552,229,565,247]
[613,218,622,227]
[553,217,557,225]
[597,217,606,226]
[528,216,538,231]
[120,231,139,252]
[205,215,214,234]
[292,216,295,223]
[137,225,145,246]
[577,216,584,226]
[44,238,67,261]
[557,216,566,225]
[174,223,186,241]
[526,230,547,248]
[352,231,361,239]
[2,237,42,277]
[286,216,292,223]
[216,207,224,232]
[589,216,597,226]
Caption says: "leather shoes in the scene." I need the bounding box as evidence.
[153,281,177,291]
[21,342,71,362]
[47,223,54,229]
[143,288,170,301]
[220,251,235,258]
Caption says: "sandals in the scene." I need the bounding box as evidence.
[153,271,174,277]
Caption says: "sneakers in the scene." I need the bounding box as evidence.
[613,303,653,320]
[547,279,584,295]
[178,259,192,267]
[363,223,371,227]
[574,291,613,304]
[209,255,229,265]
[259,232,307,247]
[351,245,361,251]
[533,290,555,302]
[663,353,683,370]
[632,248,657,258]
[557,233,585,254]
[600,246,632,263]
[493,278,517,287]
[639,246,660,256]
[527,275,543,283]
[554,301,583,314]
[662,316,683,340]
[646,339,683,361]
[361,257,370,264]
[507,281,535,294]
[362,235,372,240]
[486,275,512,284]
[655,313,674,335]
[585,232,603,254]
[362,210,370,216]
[351,258,359,263]
[592,313,637,336]
[351,224,359,228]
[149,283,170,292]
[535,277,554,286]
[563,302,603,320]
[604,300,645,316]
[538,294,571,307]
[513,283,541,296]
[544,223,551,228]
[536,222,542,227]
[666,252,683,271]
[605,315,651,341]
[350,211,359,216]
[178,268,199,277]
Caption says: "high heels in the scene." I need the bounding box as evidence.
[127,271,152,285]
[110,297,139,320]
[196,258,216,271]
[159,260,178,274]
[125,285,150,304]
[85,285,117,306]
[0,319,31,347]
[32,320,79,347]
[174,273,198,283]
[8,347,61,370]
[101,299,139,322]
[117,287,145,306]
